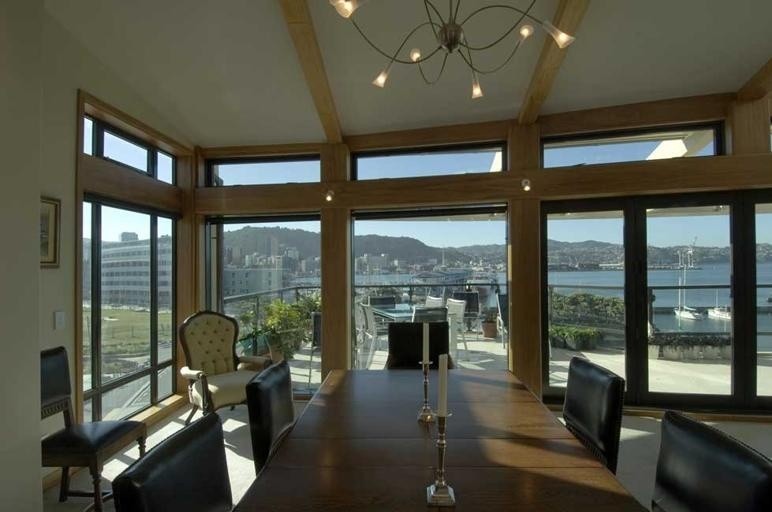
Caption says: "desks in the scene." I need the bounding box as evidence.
[218,363,651,510]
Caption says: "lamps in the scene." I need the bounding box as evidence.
[325,1,577,100]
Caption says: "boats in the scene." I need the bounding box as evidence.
[707,306,731,321]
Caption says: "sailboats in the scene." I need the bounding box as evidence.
[673,264,706,320]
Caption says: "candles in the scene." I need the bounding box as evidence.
[437,352,448,414]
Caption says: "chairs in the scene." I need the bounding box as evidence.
[106,411,236,511]
[39,342,149,511]
[648,399,771,512]
[300,283,510,384]
[174,309,274,427]
[550,352,625,478]
[241,357,297,480]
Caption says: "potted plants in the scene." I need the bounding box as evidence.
[561,327,601,350]
[546,323,575,350]
[267,339,295,364]
[234,292,323,359]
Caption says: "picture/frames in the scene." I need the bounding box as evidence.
[39,194,62,270]
